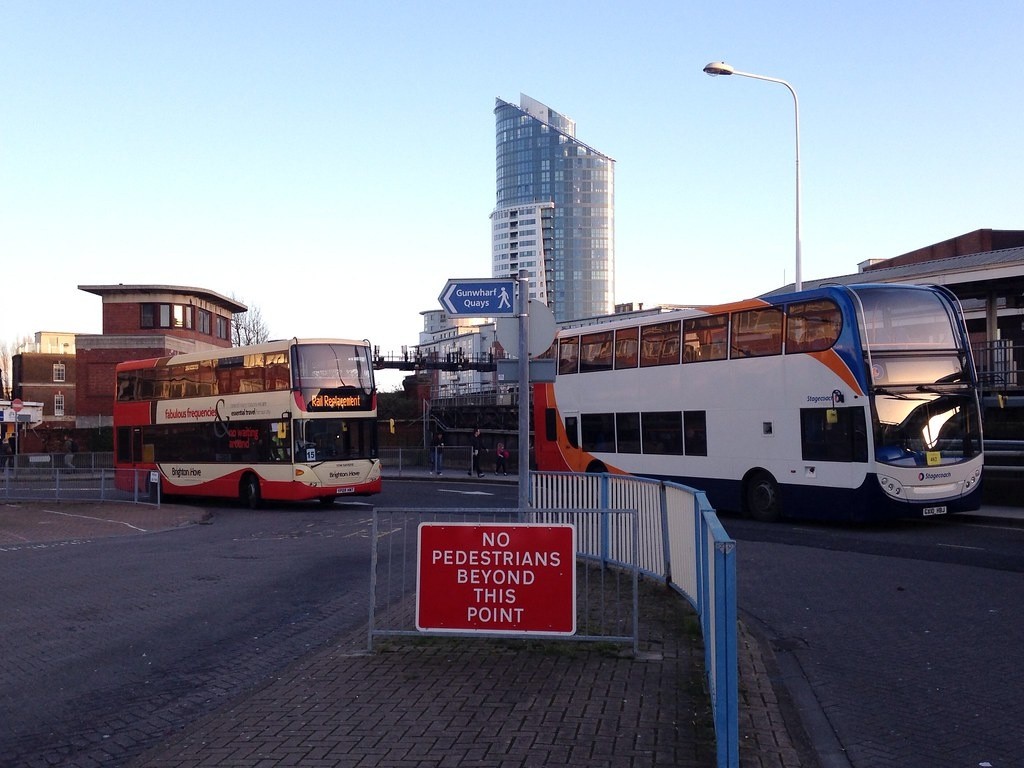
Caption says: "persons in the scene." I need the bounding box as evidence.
[0,432,20,472]
[494,443,508,477]
[429,431,444,476]
[467,426,488,478]
[63,434,76,472]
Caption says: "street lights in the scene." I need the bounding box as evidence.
[702,61,802,292]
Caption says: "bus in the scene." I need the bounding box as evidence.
[111,337,382,508]
[555,282,1010,523]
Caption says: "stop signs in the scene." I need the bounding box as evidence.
[12,399,23,412]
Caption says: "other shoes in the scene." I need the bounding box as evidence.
[438,472,442,475]
[430,472,432,474]
[495,471,498,475]
[479,474,485,478]
[504,473,507,476]
[468,471,471,475]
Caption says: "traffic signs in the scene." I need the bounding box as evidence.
[438,277,518,318]
